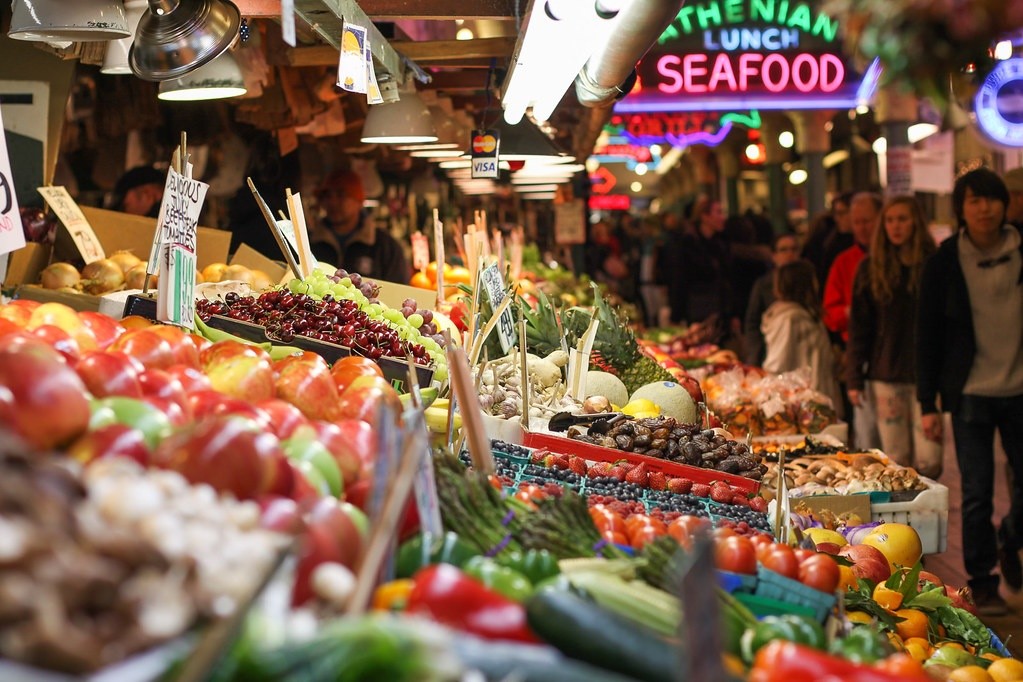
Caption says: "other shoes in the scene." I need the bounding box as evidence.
[997,518,1023,591]
[968,574,1007,615]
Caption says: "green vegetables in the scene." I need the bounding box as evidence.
[524,243,603,294]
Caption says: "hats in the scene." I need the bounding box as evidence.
[311,168,364,208]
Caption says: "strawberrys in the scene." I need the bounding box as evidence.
[533,446,766,513]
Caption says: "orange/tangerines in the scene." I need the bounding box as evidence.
[410,260,578,307]
[702,373,829,438]
[791,523,1023,682]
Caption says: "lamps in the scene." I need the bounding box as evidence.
[157,51,247,101]
[5,0,131,40]
[499,0,620,125]
[98,0,149,74]
[361,72,585,200]
[127,0,242,82]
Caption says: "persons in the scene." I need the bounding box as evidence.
[566,179,943,564]
[59,75,559,281]
[933,168,1023,617]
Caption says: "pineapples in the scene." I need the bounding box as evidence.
[588,358,677,393]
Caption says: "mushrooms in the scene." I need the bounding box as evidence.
[765,457,931,493]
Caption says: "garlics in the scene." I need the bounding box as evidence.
[470,361,582,433]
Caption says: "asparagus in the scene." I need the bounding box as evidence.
[435,451,759,655]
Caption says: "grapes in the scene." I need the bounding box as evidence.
[291,264,456,383]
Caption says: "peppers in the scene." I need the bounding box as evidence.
[381,533,914,682]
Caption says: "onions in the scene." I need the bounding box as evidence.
[41,253,275,294]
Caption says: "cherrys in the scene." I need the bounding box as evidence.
[196,280,430,366]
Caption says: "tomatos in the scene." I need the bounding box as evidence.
[513,486,839,594]
[0,295,419,602]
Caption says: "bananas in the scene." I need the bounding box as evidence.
[183,315,306,360]
[398,387,463,449]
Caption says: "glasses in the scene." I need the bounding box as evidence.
[775,245,798,253]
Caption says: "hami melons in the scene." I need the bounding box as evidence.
[578,372,696,426]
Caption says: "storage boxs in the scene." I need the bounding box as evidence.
[0,201,1011,682]
